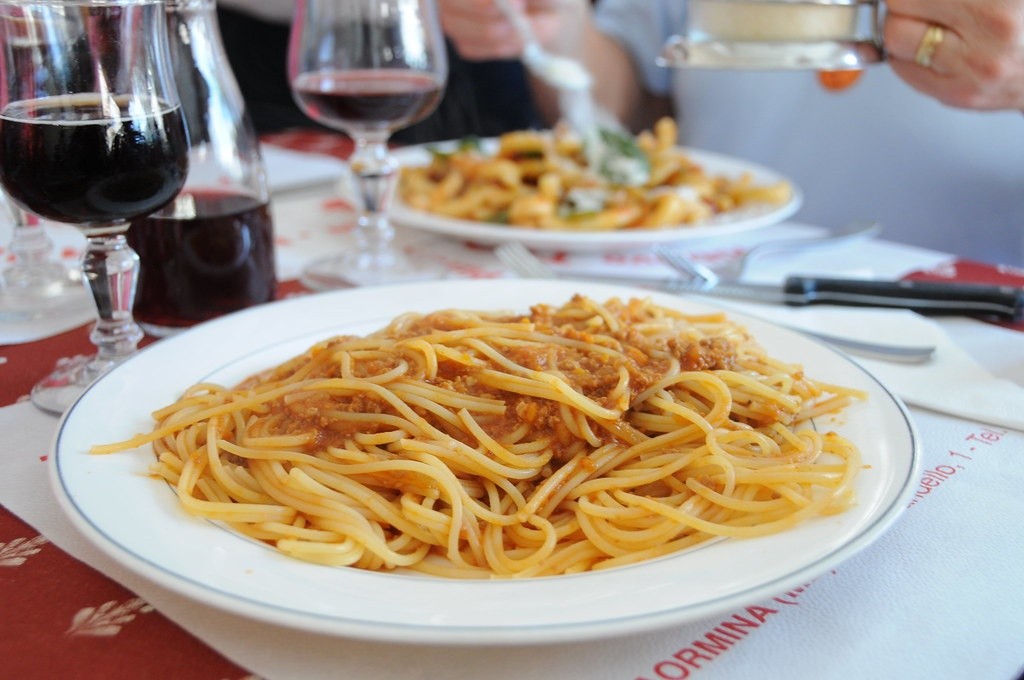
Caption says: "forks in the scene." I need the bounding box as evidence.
[653,218,882,284]
[495,238,561,281]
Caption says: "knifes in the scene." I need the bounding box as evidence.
[558,266,1024,314]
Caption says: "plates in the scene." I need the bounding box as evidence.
[686,288,940,357]
[48,278,923,650]
[335,131,805,255]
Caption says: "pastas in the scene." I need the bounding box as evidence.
[394,113,784,227]
[86,294,869,580]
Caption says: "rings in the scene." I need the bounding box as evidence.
[915,23,944,68]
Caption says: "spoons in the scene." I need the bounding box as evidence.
[496,2,593,91]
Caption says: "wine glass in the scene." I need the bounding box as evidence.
[0,0,191,416]
[284,0,453,292]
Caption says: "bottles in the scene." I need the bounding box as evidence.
[118,1,279,337]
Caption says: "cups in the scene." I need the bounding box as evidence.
[652,0,893,71]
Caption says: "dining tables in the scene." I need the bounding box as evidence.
[0,129,1024,680]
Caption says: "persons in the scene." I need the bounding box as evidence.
[67,1,641,141]
[883,0,1024,111]
